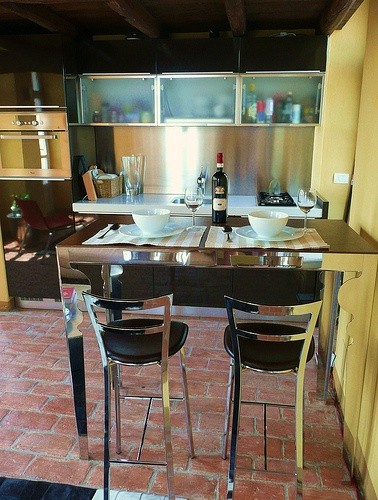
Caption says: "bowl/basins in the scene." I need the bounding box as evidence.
[249,211,288,238]
[132,209,170,235]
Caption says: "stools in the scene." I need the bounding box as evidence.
[221,295,322,500]
[82,291,195,500]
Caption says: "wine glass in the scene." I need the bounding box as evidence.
[184,187,203,217]
[296,187,317,233]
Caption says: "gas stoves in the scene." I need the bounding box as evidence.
[257,190,297,208]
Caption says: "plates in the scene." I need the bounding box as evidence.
[118,224,184,237]
[235,225,304,241]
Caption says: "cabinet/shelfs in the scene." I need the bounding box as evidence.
[76,70,326,127]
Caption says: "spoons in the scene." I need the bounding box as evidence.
[98,223,120,238]
[221,225,233,243]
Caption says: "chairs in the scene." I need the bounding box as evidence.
[13,197,73,264]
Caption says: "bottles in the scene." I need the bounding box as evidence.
[91,93,152,123]
[241,83,318,125]
[212,152,229,225]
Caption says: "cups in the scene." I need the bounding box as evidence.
[121,155,143,196]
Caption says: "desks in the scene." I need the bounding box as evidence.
[55,217,378,485]
